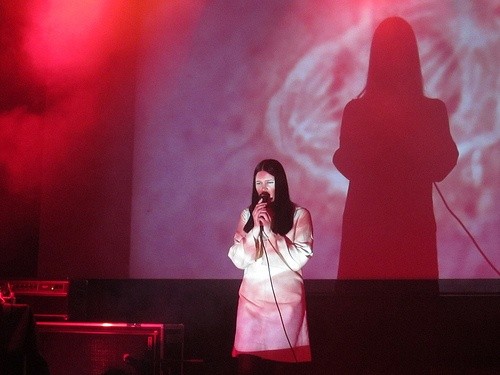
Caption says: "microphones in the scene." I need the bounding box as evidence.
[259,191,270,231]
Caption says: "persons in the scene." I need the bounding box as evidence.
[227,158,316,375]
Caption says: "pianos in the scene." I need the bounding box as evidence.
[0,279,89,298]
